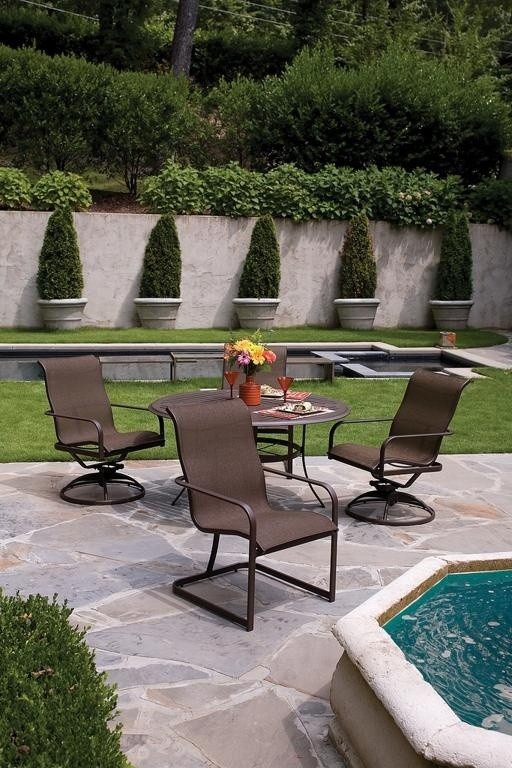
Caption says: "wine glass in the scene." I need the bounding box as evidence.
[276,376,295,406]
[223,371,240,402]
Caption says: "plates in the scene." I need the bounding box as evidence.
[261,389,290,396]
[275,403,328,414]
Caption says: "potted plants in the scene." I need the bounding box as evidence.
[37,212,89,332]
[332,211,380,331]
[428,207,475,331]
[132,216,183,328]
[232,215,281,331]
